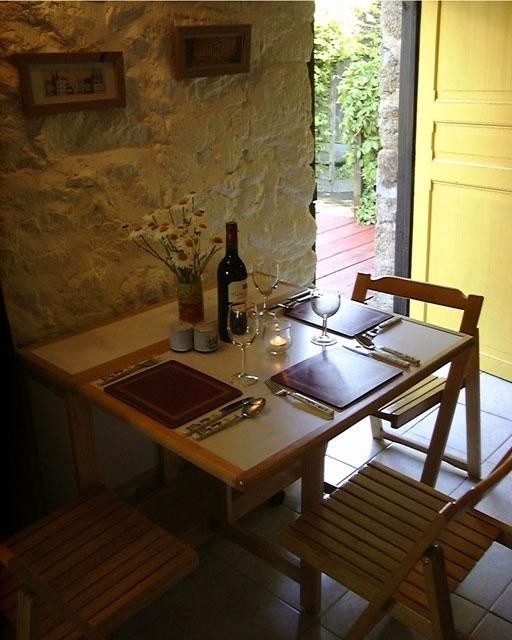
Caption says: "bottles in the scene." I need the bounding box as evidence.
[169,321,194,353]
[193,322,219,353]
[217,221,248,344]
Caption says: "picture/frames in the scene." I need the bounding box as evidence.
[177,24,250,79]
[18,50,126,115]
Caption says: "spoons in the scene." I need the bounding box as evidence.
[193,396,266,441]
[354,334,420,366]
[277,293,316,309]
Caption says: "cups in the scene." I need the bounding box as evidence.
[263,319,293,356]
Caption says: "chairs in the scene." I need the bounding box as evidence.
[0,482,200,640]
[275,446,512,640]
[352,272,482,488]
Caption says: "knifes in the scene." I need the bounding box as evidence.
[180,397,255,437]
[267,288,311,311]
[342,344,411,367]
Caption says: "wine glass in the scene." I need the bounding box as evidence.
[227,302,258,385]
[252,259,279,318]
[311,287,340,346]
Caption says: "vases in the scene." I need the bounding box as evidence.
[177,280,206,324]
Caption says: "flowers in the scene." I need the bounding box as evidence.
[119,191,223,279]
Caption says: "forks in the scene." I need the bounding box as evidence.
[265,378,334,416]
[365,315,401,340]
